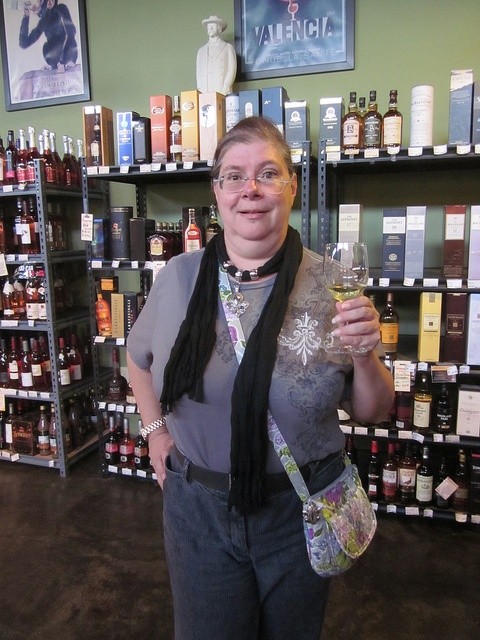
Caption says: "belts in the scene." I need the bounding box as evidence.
[174,445,341,493]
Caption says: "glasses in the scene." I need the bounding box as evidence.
[212,171,295,195]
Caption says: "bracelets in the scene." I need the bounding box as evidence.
[138,417,167,442]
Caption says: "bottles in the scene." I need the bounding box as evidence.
[89,389,100,433]
[0,138,4,186]
[52,267,78,315]
[184,208,202,253]
[175,223,183,255]
[396,391,412,431]
[162,222,173,261]
[50,408,57,456]
[11,197,23,254]
[365,440,381,503]
[433,383,455,434]
[36,406,50,455]
[37,269,47,321]
[80,390,90,434]
[29,338,34,357]
[169,96,182,162]
[147,221,170,261]
[41,128,54,184]
[342,92,363,148]
[68,398,83,447]
[3,403,15,451]
[60,403,73,453]
[31,339,47,391]
[398,440,417,505]
[68,137,80,186]
[82,324,94,377]
[37,134,44,156]
[452,449,470,512]
[50,403,54,407]
[0,411,5,449]
[4,129,18,184]
[69,334,83,381]
[49,133,62,184]
[21,335,32,390]
[89,113,102,167]
[24,269,39,320]
[0,206,10,254]
[168,222,178,257]
[19,200,36,254]
[8,336,20,389]
[117,419,134,466]
[434,448,451,510]
[205,204,222,247]
[46,199,67,252]
[357,96,366,118]
[25,126,40,184]
[16,128,27,185]
[61,135,78,186]
[104,416,118,465]
[381,441,398,505]
[15,138,19,151]
[40,337,52,391]
[412,371,432,434]
[97,386,105,399]
[382,89,402,149]
[133,418,149,469]
[94,282,112,337]
[0,339,9,388]
[13,272,26,320]
[29,197,41,254]
[379,292,399,361]
[76,139,84,164]
[125,380,136,405]
[16,400,23,415]
[3,266,18,319]
[345,434,353,462]
[415,446,433,508]
[57,337,72,386]
[362,91,381,148]
[106,346,127,401]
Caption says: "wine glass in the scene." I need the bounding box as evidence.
[319,239,371,357]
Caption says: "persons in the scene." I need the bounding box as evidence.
[192,13,239,96]
[129,115,394,633]
[12,1,81,76]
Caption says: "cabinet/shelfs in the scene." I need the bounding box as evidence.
[316,138,479,525]
[0,159,113,478]
[80,141,311,480]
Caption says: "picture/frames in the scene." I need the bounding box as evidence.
[235,1,353,82]
[0,1,91,112]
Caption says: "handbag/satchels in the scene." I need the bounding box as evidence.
[302,454,378,579]
